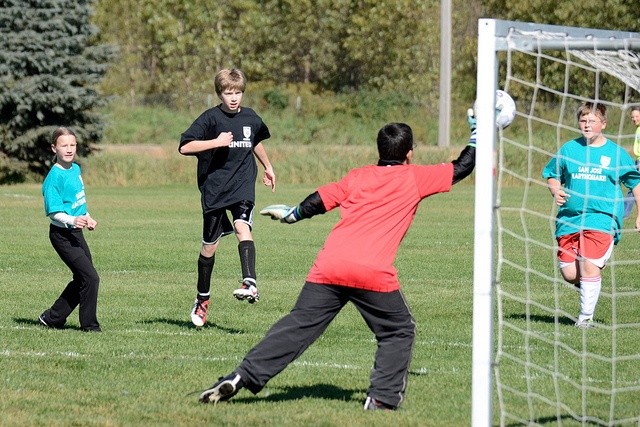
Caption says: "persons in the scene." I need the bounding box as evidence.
[38,126,101,331]
[542,102,640,329]
[199,109,476,410]
[622,106,640,221]
[178,68,276,327]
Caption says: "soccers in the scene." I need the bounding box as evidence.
[473,89,515,131]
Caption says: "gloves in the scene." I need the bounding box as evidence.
[260,204,296,224]
[468,103,503,147]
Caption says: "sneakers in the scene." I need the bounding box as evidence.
[575,314,597,323]
[191,299,209,325]
[39,311,57,328]
[233,285,259,304]
[363,397,395,412]
[198,373,243,403]
